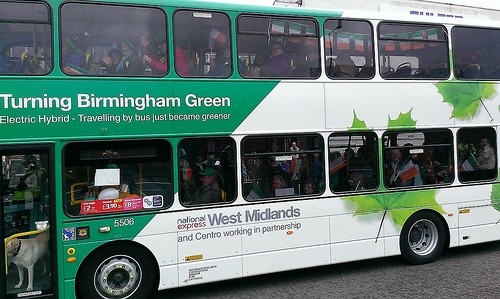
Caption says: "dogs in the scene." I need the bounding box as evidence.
[5,228,51,292]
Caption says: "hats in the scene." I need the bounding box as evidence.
[269,36,286,49]
[198,166,218,176]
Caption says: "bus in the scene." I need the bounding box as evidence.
[0,0,500,299]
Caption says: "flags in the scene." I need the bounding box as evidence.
[459,153,481,172]
[397,158,417,183]
[329,157,345,174]
[208,29,229,48]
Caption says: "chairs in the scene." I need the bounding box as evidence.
[326,58,500,77]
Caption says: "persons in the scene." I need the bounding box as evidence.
[3,33,494,231]
